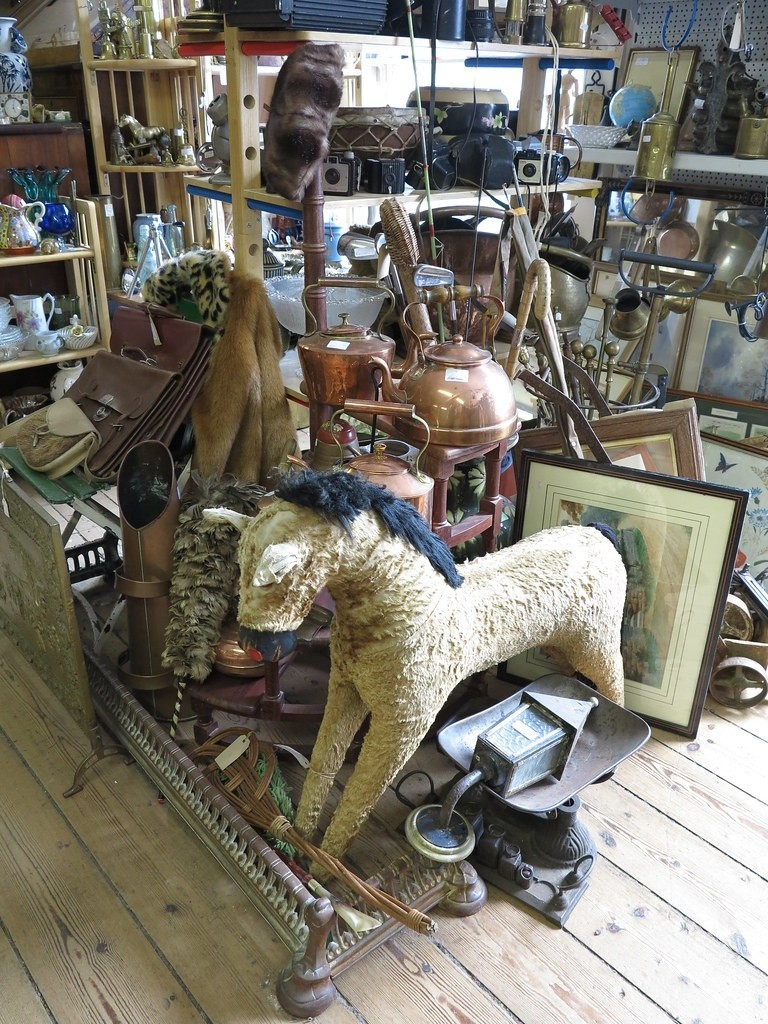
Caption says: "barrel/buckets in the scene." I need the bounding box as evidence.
[324,213,341,262]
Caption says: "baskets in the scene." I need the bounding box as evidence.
[564,124,628,149]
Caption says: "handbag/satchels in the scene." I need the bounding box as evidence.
[59,347,184,476]
[110,306,216,444]
[16,397,119,482]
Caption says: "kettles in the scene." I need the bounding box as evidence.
[298,277,438,406]
[368,284,521,447]
[331,398,434,534]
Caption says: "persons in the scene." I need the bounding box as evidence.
[558,69,579,129]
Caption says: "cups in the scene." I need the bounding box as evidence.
[35,331,65,355]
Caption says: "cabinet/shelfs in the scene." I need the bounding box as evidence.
[30,62,156,256]
[195,52,369,253]
[174,15,620,452]
[0,195,111,423]
[74,0,204,319]
[0,120,92,397]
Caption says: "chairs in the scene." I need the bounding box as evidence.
[0,250,300,656]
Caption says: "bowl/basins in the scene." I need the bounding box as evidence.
[0,332,29,360]
[58,325,98,349]
[263,272,389,336]
[568,125,627,148]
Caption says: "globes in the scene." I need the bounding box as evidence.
[608,84,658,128]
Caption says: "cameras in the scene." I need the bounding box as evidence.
[322,133,570,196]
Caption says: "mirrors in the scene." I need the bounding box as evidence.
[586,178,768,298]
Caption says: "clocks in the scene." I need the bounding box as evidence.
[0,92,33,124]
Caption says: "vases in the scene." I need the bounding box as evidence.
[0,17,33,93]
[133,214,164,252]
[328,108,426,170]
[50,359,84,402]
[7,165,72,242]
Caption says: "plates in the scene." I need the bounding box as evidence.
[0,297,23,343]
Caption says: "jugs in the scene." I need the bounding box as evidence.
[0,201,45,256]
[9,293,54,350]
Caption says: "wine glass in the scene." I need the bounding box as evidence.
[41,203,76,253]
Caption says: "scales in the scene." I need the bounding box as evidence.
[440,672,651,930]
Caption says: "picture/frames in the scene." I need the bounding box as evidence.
[473,0,508,13]
[510,406,705,492]
[674,292,768,405]
[621,46,700,123]
[623,385,768,448]
[579,354,637,420]
[592,261,727,388]
[700,431,768,595]
[580,294,644,370]
[497,448,751,739]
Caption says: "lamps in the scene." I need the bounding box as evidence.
[404,690,598,864]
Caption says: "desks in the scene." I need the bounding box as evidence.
[348,382,519,556]
[563,146,768,186]
